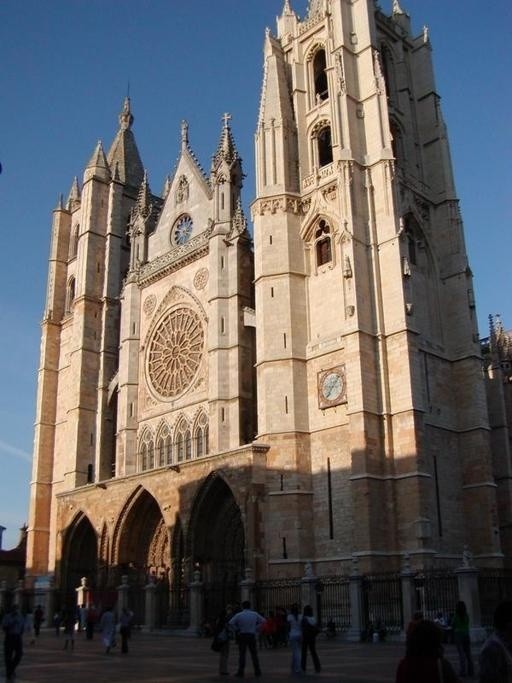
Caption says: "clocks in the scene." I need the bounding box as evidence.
[316,363,348,410]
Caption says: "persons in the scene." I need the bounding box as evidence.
[405,612,424,639]
[432,611,449,643]
[370,622,384,641]
[478,600,512,683]
[33,603,134,657]
[199,600,322,678]
[447,600,474,677]
[325,617,337,640]
[2,602,26,683]
[395,619,462,682]
